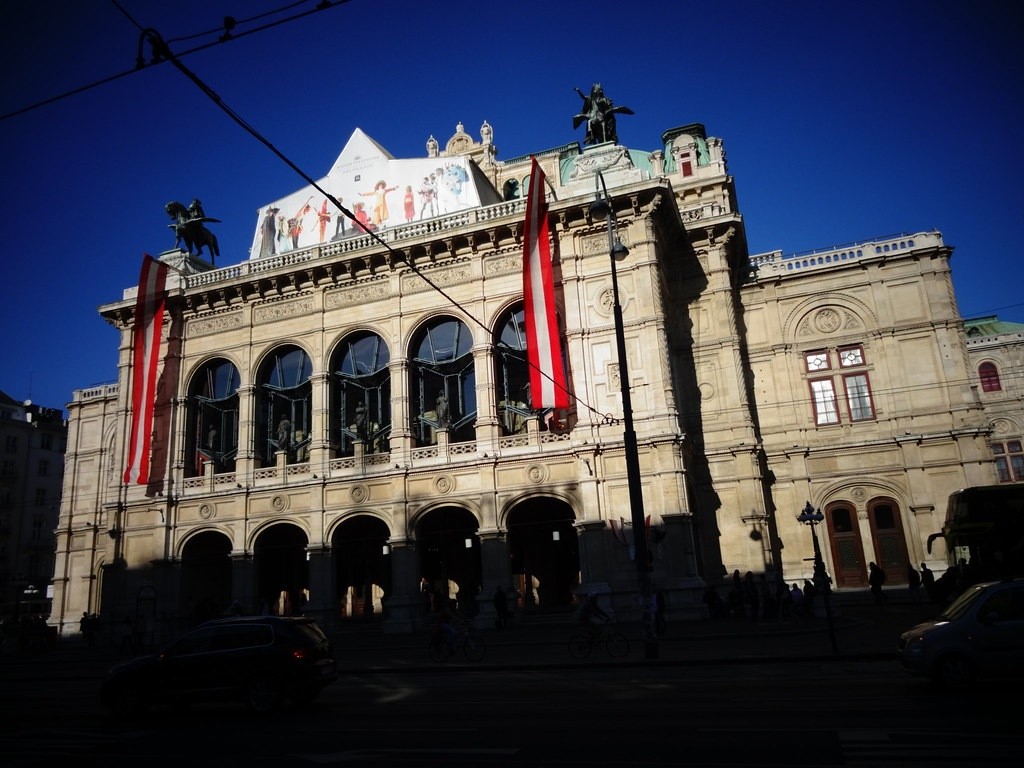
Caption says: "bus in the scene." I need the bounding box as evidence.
[927,483,1024,596]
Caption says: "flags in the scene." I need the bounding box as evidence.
[524,158,569,409]
[125,256,169,480]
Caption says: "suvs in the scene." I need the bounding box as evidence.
[105,615,335,716]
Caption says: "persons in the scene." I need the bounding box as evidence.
[577,592,614,650]
[867,562,885,596]
[906,562,934,604]
[491,585,509,630]
[180,198,206,257]
[258,164,460,261]
[701,562,834,624]
[79,611,99,648]
[120,612,147,656]
[432,599,467,657]
[572,79,616,143]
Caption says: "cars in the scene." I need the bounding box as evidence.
[893,578,1023,690]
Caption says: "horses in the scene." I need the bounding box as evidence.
[572,83,635,143]
[165,201,221,266]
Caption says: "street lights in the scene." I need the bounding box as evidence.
[590,168,654,623]
[796,501,842,655]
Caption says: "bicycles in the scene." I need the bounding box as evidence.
[428,627,487,664]
[567,611,630,660]
